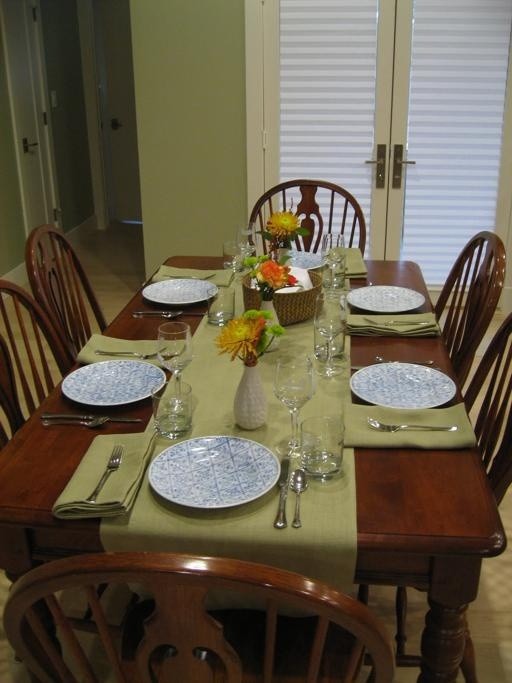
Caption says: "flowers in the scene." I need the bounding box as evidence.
[244,253,303,298]
[254,207,309,250]
[214,309,284,363]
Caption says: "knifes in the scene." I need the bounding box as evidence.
[350,365,442,372]
[128,307,212,318]
[40,412,143,424]
[274,457,292,531]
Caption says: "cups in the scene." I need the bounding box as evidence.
[205,286,236,326]
[323,255,346,289]
[300,416,345,479]
[222,241,242,272]
[314,310,345,362]
[151,381,195,440]
[236,223,258,261]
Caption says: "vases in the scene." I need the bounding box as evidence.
[257,299,281,351]
[275,248,288,264]
[233,362,267,430]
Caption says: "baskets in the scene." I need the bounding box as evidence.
[242,269,323,326]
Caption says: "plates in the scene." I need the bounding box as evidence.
[61,360,166,405]
[142,278,218,306]
[349,363,457,409]
[346,284,427,313]
[147,435,279,511]
[284,251,325,270]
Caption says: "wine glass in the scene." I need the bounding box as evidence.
[313,291,345,376]
[157,321,193,400]
[321,233,347,299]
[272,353,314,458]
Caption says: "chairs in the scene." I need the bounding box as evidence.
[26,222,106,358]
[0,552,394,683]
[345,314,512,683]
[248,180,366,257]
[0,280,72,452]
[433,232,506,390]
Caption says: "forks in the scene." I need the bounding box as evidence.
[96,346,168,358]
[164,272,216,280]
[85,443,124,502]
[366,416,458,434]
[363,316,429,326]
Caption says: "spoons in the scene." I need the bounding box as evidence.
[127,310,184,320]
[289,469,307,529]
[373,354,435,366]
[40,417,112,430]
